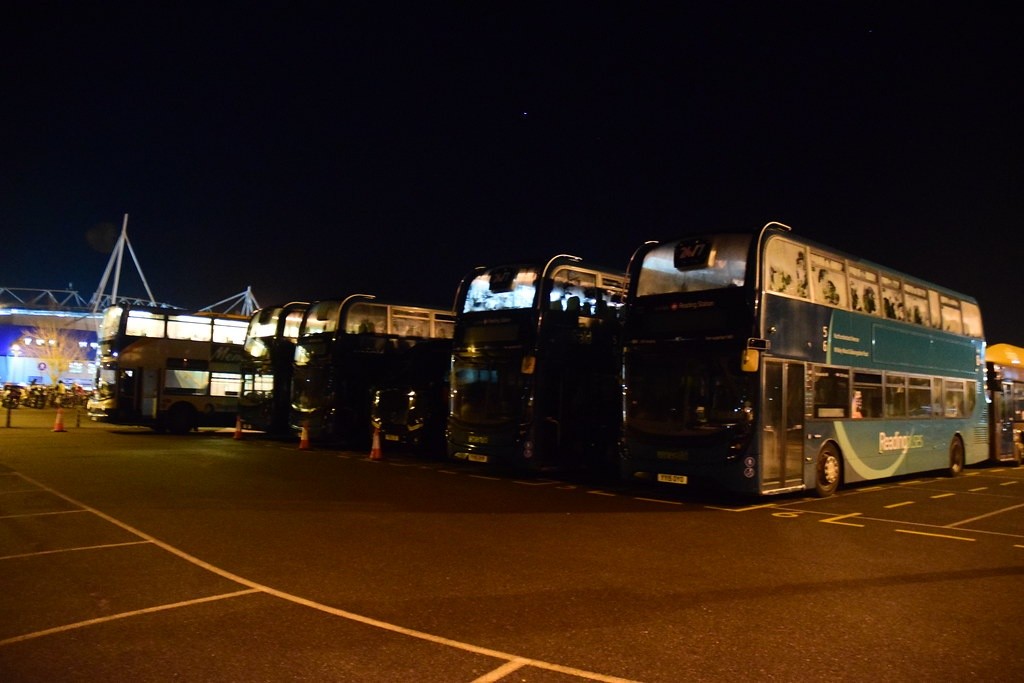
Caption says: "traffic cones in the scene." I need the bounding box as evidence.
[50,408,68,433]
[232,415,243,440]
[299,422,312,451]
[369,428,383,461]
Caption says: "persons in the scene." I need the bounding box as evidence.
[30,379,37,385]
[71,383,87,408]
[55,380,66,408]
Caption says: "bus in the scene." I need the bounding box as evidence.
[238,297,320,441]
[85,300,251,437]
[985,342,1024,467]
[287,294,456,460]
[439,252,629,484]
[609,218,991,503]
